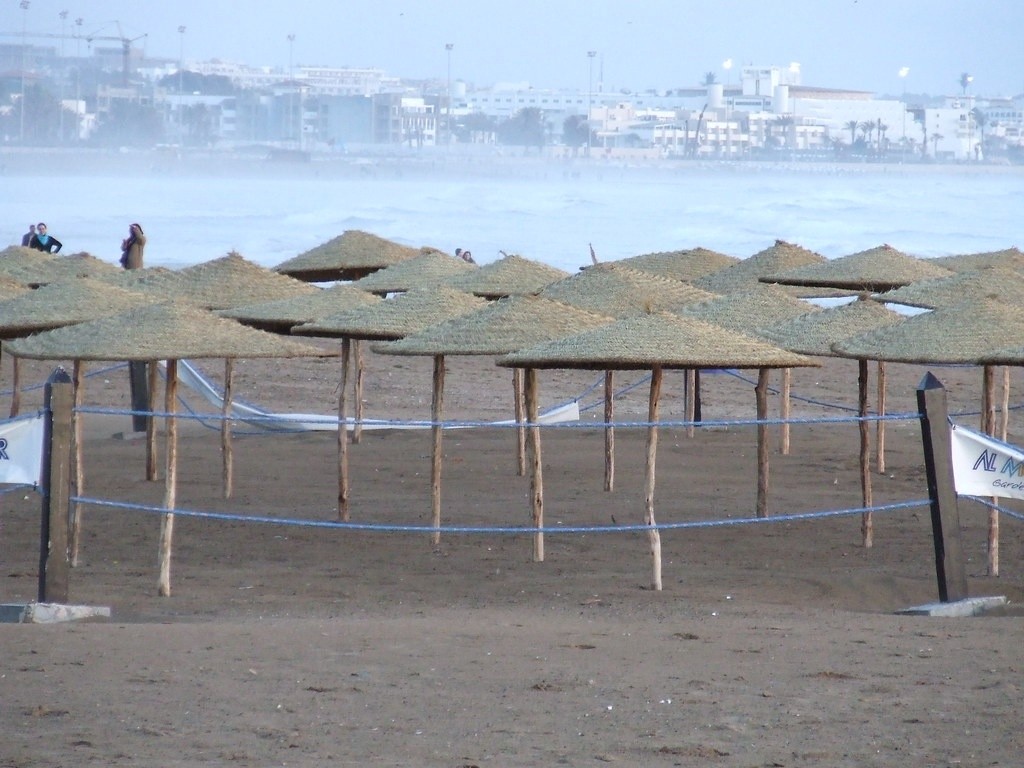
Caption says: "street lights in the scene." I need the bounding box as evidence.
[444,42,456,94]
[74,17,84,53]
[19,0,31,46]
[174,24,187,59]
[588,49,597,103]
[58,9,68,52]
[288,33,297,67]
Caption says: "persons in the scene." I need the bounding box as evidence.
[455,248,464,257]
[116,223,146,271]
[463,251,476,264]
[19,223,62,255]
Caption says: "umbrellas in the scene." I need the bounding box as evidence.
[0,230,1024,598]
